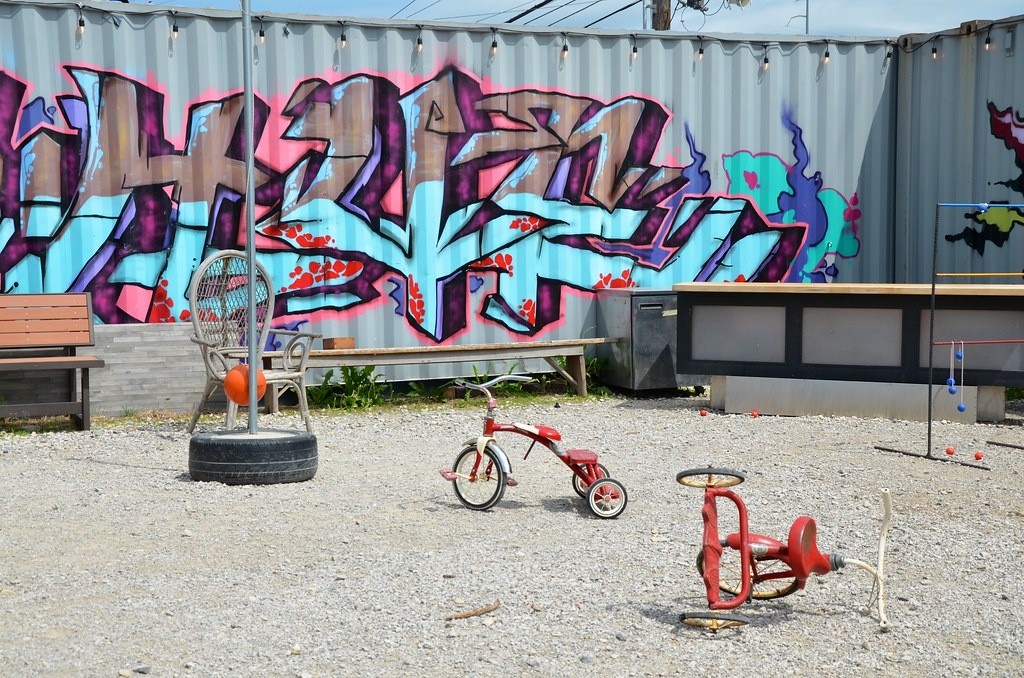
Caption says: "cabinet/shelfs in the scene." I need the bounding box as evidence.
[594,286,709,395]
[672,281,1023,426]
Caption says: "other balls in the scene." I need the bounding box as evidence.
[945,351,984,460]
[224,364,266,406]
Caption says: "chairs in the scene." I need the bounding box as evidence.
[186,249,315,437]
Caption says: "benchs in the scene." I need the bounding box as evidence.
[227,334,621,415]
[0,291,106,432]
